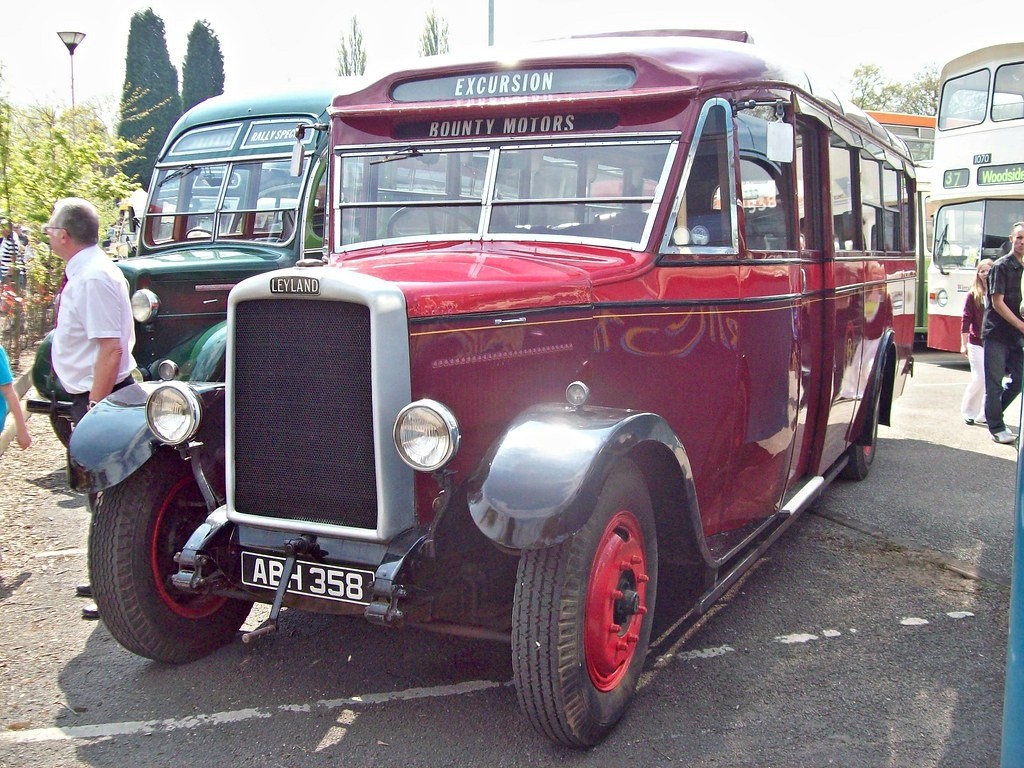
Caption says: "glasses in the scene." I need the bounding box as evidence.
[42,224,70,237]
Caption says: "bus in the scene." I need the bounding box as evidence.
[24,86,627,448]
[927,42,1020,351]
[64,31,924,748]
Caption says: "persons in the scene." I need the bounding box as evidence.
[960,222,1024,444]
[0,217,51,334]
[0,344,30,451]
[41,197,138,599]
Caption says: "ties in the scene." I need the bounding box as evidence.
[50,273,69,377]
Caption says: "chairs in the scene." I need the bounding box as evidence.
[625,202,870,255]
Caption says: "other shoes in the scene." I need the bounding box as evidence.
[76,583,92,596]
[993,427,1018,443]
[964,419,974,425]
[83,603,99,617]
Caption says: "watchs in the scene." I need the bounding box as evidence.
[86,400,97,409]
